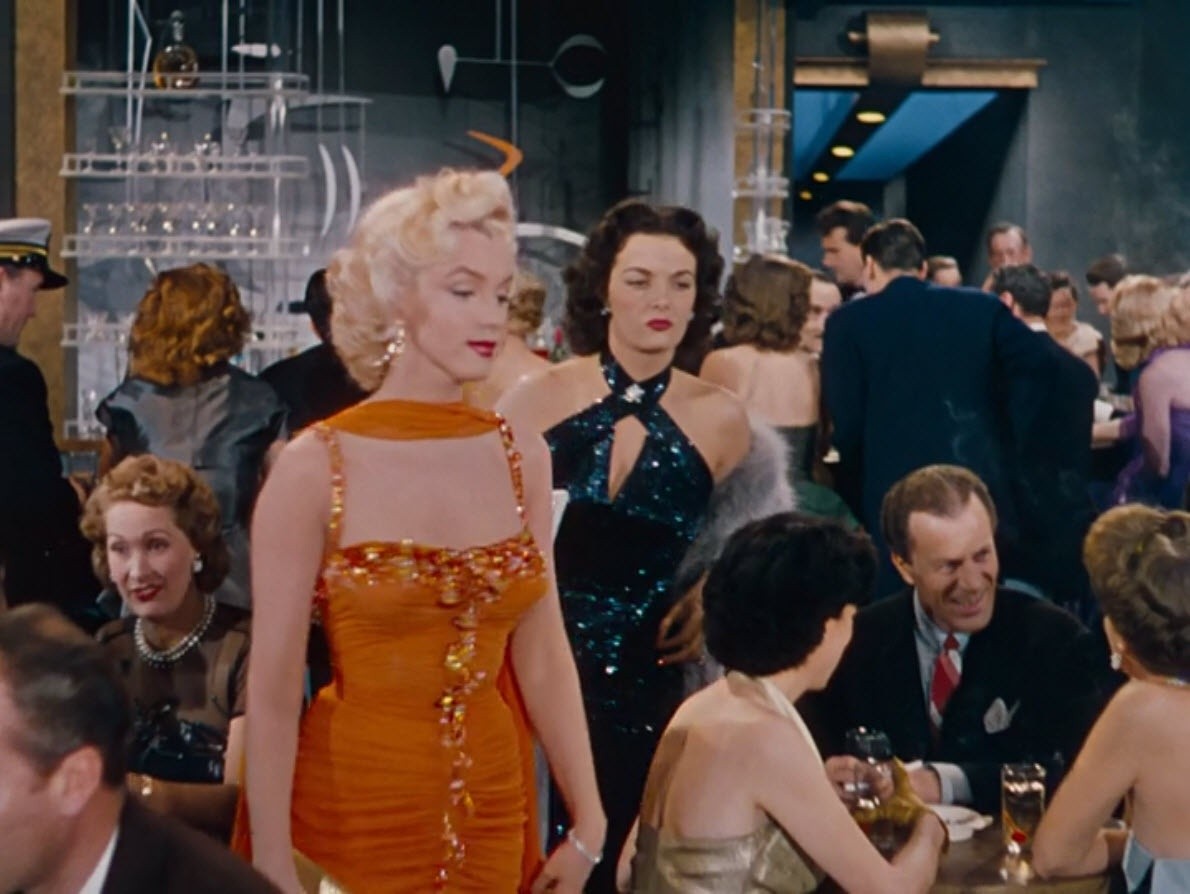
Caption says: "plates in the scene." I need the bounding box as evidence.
[930,803,984,841]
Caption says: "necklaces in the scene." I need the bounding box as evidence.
[132,591,218,663]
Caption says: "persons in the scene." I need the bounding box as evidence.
[0,166,1190,894]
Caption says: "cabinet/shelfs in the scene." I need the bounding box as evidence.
[55,67,308,455]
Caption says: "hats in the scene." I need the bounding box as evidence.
[0,218,69,291]
[277,269,330,312]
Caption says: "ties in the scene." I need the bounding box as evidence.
[930,635,962,751]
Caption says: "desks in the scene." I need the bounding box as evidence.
[826,816,1111,894]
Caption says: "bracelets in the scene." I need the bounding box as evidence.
[567,829,605,864]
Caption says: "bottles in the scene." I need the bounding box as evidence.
[154,11,197,89]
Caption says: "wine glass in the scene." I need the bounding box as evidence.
[107,125,128,170]
[80,200,270,254]
[226,127,246,170]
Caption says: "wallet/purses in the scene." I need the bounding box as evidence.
[292,848,346,894]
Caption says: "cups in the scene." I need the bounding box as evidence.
[193,141,220,171]
[854,730,893,811]
[1002,763,1047,884]
[148,140,178,173]
